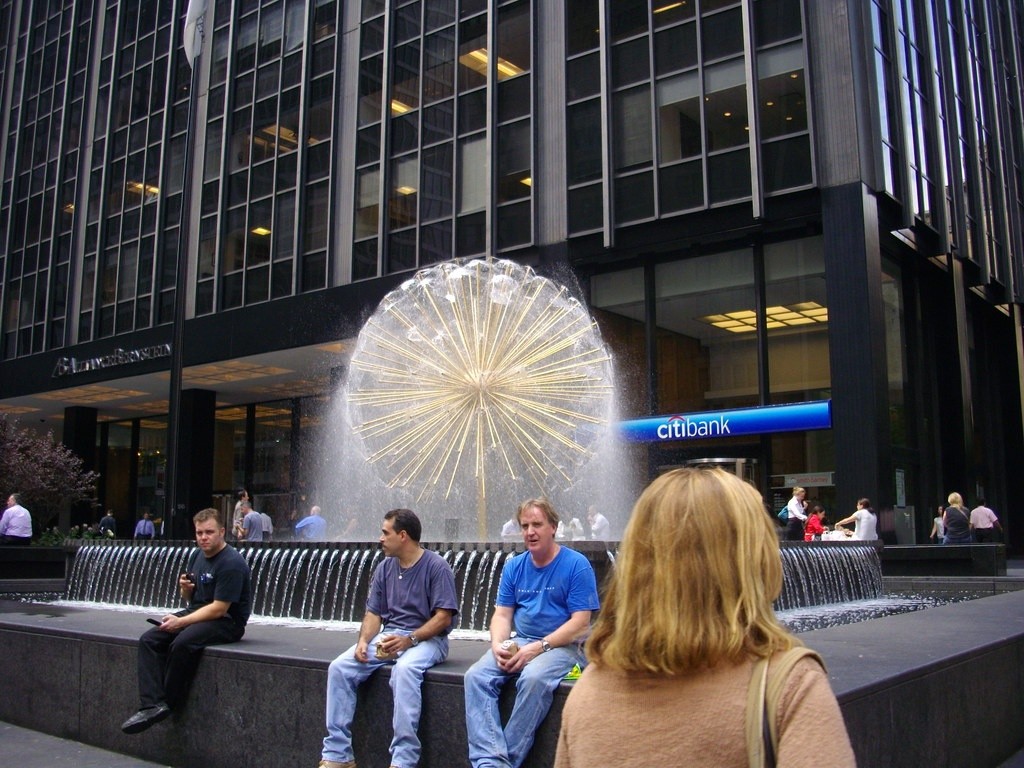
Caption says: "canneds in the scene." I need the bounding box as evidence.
[501,640,518,660]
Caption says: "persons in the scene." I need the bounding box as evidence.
[295,506,362,542]
[784,486,808,541]
[554,467,856,768]
[804,505,829,541]
[0,494,32,546]
[232,489,273,542]
[930,505,948,545]
[121,508,251,734]
[943,492,970,546]
[969,498,1002,543]
[835,498,878,541]
[155,483,164,499]
[500,515,522,542]
[134,512,155,540]
[555,505,611,541]
[319,509,458,768]
[463,496,599,768]
[97,510,116,540]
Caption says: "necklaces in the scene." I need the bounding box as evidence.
[396,558,422,585]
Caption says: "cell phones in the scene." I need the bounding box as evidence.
[147,618,164,626]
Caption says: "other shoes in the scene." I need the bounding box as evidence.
[318,759,357,768]
[122,703,171,734]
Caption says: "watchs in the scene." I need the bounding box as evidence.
[407,634,418,647]
[539,639,550,652]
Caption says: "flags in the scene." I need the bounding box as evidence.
[183,0,207,69]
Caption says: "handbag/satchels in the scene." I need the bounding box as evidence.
[777,505,788,524]
